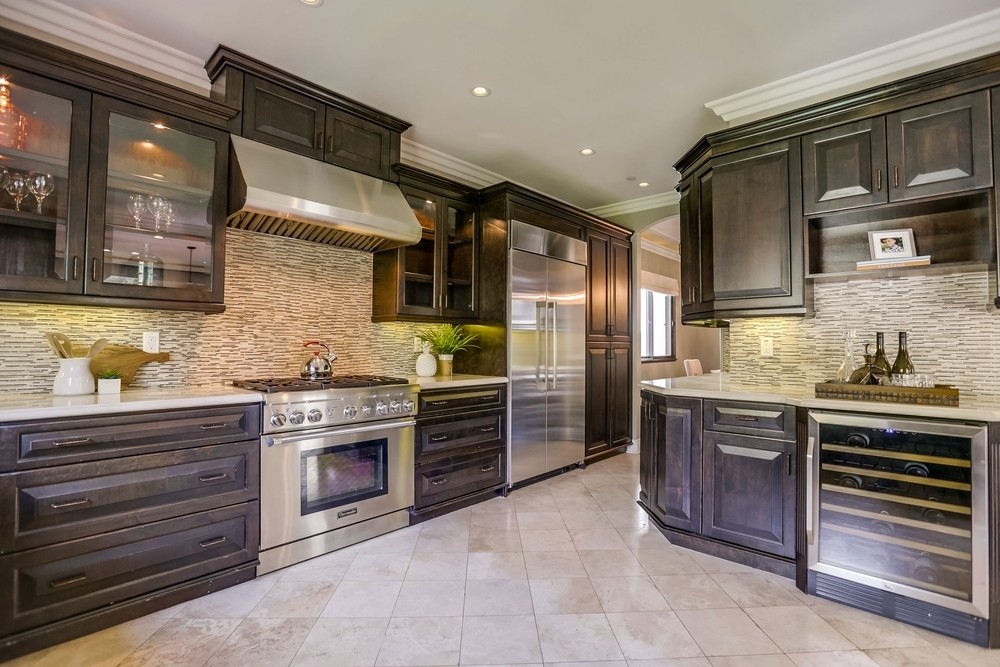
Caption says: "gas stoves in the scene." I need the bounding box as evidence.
[221,373,421,434]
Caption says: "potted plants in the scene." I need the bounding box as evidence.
[435,324,476,376]
[98,369,121,394]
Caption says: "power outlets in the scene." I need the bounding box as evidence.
[142,332,160,353]
[759,337,774,359]
[414,337,421,353]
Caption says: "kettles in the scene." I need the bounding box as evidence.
[299,341,338,380]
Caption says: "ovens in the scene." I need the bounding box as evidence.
[256,417,415,577]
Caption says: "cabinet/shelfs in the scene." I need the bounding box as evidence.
[638,392,806,591]
[203,43,413,181]
[372,164,479,323]
[0,23,242,314]
[664,50,1000,327]
[0,400,263,663]
[585,229,634,456]
[413,382,508,525]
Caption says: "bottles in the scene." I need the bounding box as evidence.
[870,540,890,570]
[870,510,894,536]
[904,462,929,478]
[846,430,871,448]
[921,497,947,526]
[838,473,863,489]
[848,332,891,386]
[891,331,915,374]
[128,243,163,286]
[53,358,95,396]
[835,341,858,384]
[910,551,941,585]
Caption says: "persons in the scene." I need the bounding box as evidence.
[881,238,903,253]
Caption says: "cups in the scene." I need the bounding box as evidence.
[0,165,10,191]
[891,374,935,388]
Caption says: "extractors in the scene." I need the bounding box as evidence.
[226,133,423,253]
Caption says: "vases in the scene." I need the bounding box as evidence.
[54,358,96,395]
[416,348,437,376]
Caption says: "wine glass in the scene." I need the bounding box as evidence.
[127,192,148,230]
[23,170,54,214]
[3,169,31,211]
[146,191,176,232]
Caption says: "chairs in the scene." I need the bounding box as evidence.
[684,359,703,376]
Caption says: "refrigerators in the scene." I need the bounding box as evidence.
[507,220,586,492]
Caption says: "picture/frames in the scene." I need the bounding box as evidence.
[868,229,917,262]
